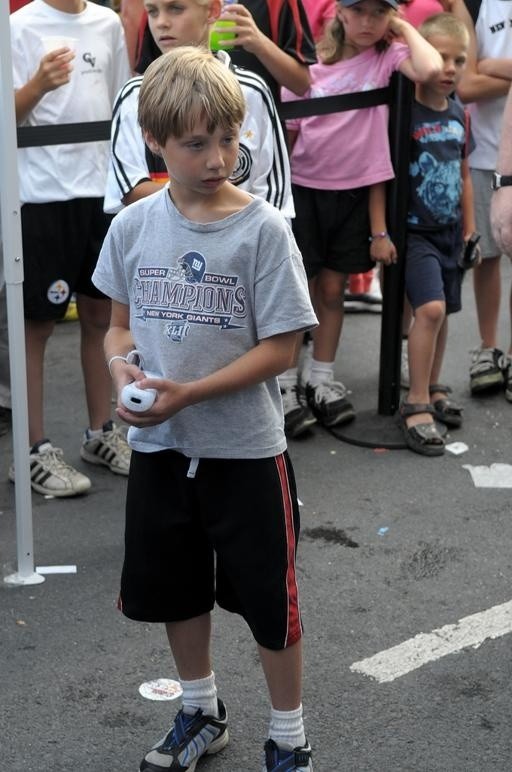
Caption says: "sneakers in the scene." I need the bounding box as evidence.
[279,385,318,438]
[498,353,512,402]
[400,339,410,388]
[8,438,91,496]
[80,419,132,476]
[305,381,356,428]
[264,719,313,772]
[140,697,229,772]
[469,348,505,395]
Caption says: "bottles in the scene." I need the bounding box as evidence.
[208,1,239,60]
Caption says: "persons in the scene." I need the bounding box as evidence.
[7,0,142,503]
[1,0,510,324]
[365,15,485,455]
[92,47,323,770]
[439,0,511,408]
[268,0,446,441]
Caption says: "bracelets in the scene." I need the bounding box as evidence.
[368,230,390,244]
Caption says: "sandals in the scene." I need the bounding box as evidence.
[430,385,464,428]
[394,394,444,456]
